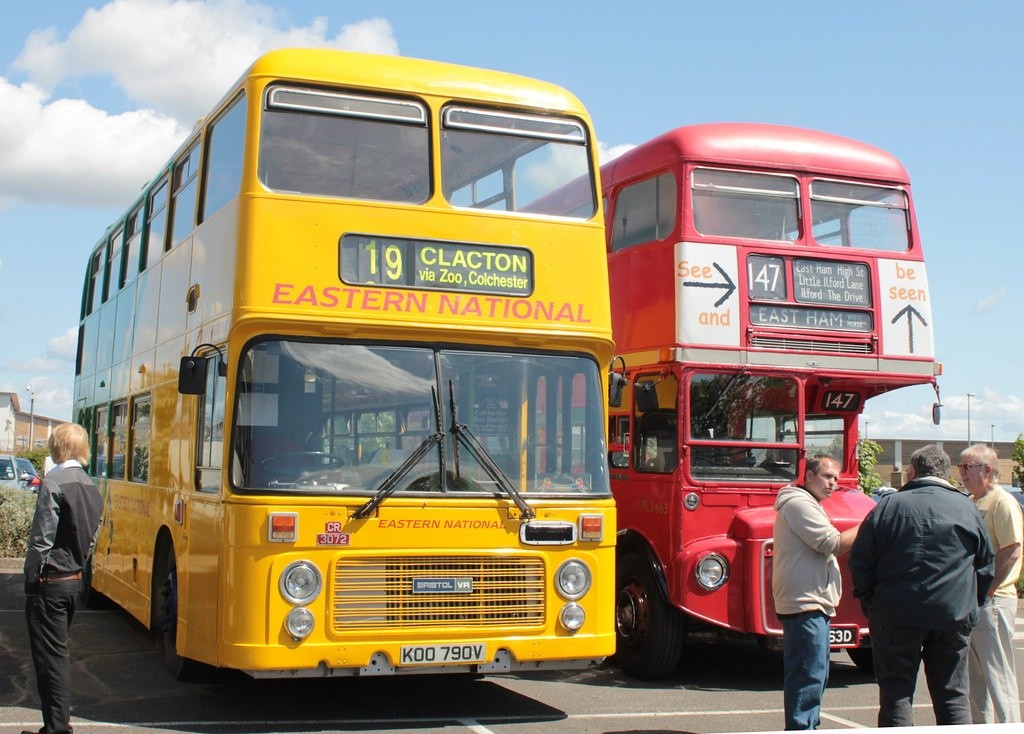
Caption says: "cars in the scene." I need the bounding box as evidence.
[0,454,42,493]
[999,484,1024,512]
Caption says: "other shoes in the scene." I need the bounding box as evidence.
[20,730,39,734]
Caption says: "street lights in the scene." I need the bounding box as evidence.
[967,391,975,447]
[991,425,995,449]
[864,421,869,443]
[25,381,35,453]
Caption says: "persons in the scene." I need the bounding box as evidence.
[954,444,1023,725]
[771,452,864,731]
[848,445,998,726]
[19,421,104,734]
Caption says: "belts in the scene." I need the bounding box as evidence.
[37,571,82,583]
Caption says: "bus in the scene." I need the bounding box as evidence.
[401,120,945,686]
[76,47,628,686]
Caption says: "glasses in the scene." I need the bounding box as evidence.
[957,463,986,470]
